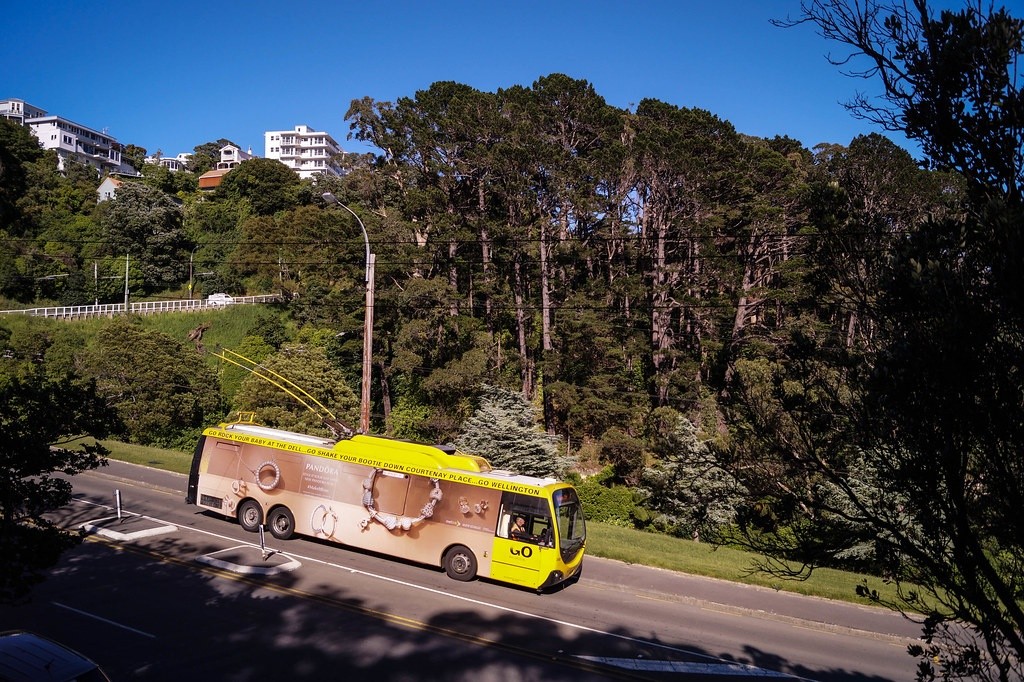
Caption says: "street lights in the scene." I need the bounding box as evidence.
[189,244,199,299]
[322,193,376,434]
[279,247,283,295]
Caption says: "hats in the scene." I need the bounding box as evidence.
[518,513,526,520]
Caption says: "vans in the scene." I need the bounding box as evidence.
[208,293,234,305]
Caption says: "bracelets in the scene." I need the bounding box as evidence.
[358,466,443,538]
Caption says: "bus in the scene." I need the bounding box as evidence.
[185,348,586,593]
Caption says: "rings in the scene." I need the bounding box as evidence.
[310,504,338,539]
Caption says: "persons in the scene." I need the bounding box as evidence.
[511,516,525,541]
